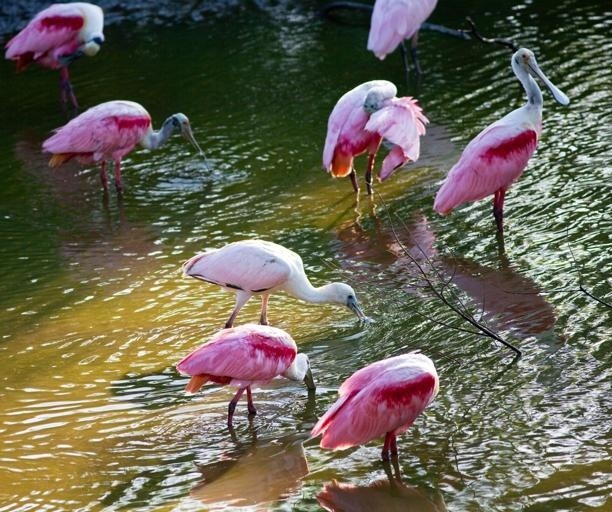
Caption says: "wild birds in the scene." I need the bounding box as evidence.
[181,237,367,329]
[37,98,202,192]
[310,348,441,458]
[432,46,570,256]
[367,0,438,74]
[440,247,572,355]
[313,456,449,512]
[0,1,106,111]
[176,321,318,428]
[321,78,430,194]
